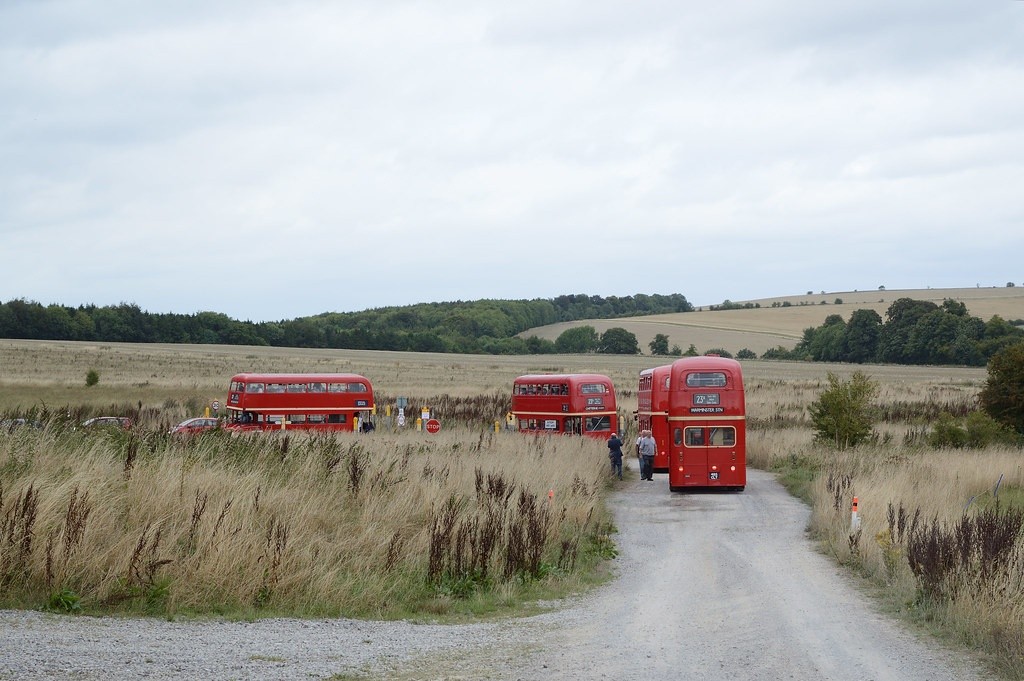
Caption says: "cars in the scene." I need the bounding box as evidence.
[4,419,43,433]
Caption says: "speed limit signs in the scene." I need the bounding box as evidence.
[212,401,219,410]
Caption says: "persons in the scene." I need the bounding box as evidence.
[520,384,599,395]
[690,372,724,385]
[607,433,623,481]
[353,412,363,432]
[196,420,202,427]
[239,382,365,393]
[528,418,602,433]
[234,412,248,422]
[636,430,658,481]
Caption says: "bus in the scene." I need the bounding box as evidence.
[511,374,618,442]
[632,364,670,468]
[225,372,375,435]
[666,353,747,492]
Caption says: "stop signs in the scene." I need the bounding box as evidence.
[425,419,441,434]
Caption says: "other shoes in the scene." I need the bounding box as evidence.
[618,479,623,481]
[641,478,646,480]
[647,478,654,481]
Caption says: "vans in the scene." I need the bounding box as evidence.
[168,417,218,436]
[82,417,133,430]
